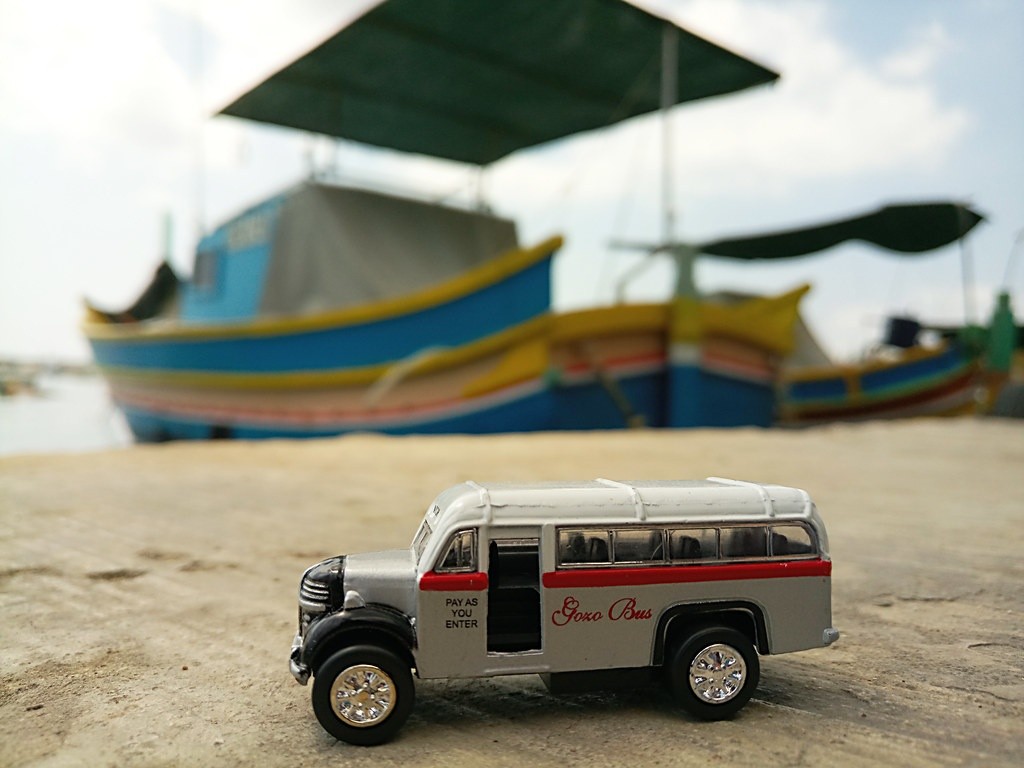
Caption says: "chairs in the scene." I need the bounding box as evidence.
[488,527,788,624]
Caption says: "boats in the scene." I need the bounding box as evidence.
[78,0,1024,435]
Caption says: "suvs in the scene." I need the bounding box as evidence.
[287,473,839,750]
